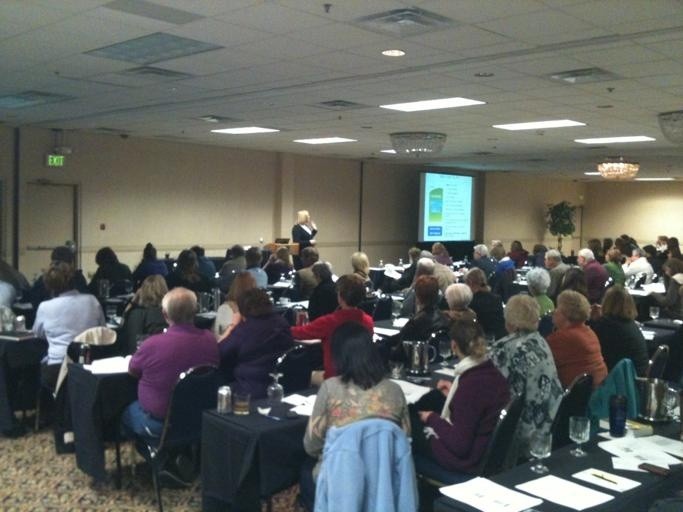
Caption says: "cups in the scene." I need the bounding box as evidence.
[279,297,291,305]
[199,292,215,312]
[98,279,109,299]
[232,392,251,415]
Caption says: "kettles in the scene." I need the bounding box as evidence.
[568,415,592,456]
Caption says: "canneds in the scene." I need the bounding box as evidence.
[216,385,233,414]
[78,344,92,364]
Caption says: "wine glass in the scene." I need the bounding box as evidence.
[649,307,659,321]
[266,373,284,403]
[439,339,451,366]
[529,429,552,475]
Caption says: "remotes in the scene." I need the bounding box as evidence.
[639,462,670,477]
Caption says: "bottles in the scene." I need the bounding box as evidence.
[398,258,403,266]
[378,260,383,268]
[609,394,627,436]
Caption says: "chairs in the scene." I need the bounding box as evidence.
[0,250,683,512]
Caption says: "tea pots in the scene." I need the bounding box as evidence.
[401,339,437,376]
[634,375,679,423]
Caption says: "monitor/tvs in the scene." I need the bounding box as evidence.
[275,238,290,243]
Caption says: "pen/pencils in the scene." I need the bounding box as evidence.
[592,473,617,484]
[266,416,281,421]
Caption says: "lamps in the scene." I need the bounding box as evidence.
[598,154,639,180]
[658,110,683,144]
[390,131,447,159]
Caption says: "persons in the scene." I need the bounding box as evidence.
[1,234,683,512]
[292,210,317,270]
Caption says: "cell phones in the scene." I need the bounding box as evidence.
[286,411,297,419]
